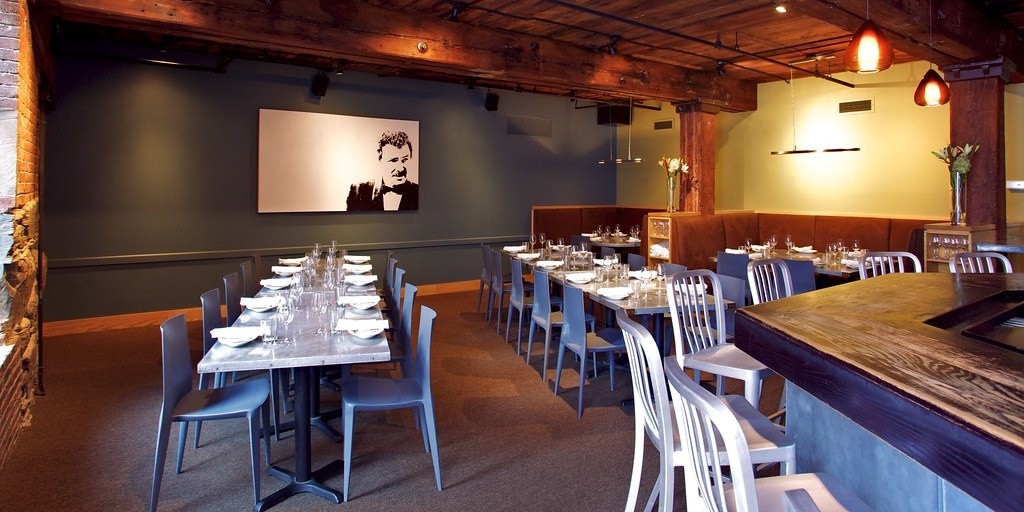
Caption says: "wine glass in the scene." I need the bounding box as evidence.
[278,240,338,346]
[929,235,969,262]
[738,235,861,270]
[529,223,670,302]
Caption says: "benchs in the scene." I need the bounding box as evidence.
[532,206,666,267]
[673,214,953,283]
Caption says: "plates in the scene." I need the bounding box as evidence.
[749,254,771,261]
[798,249,817,253]
[605,296,627,300]
[683,291,707,297]
[218,257,305,348]
[868,257,887,264]
[345,255,384,339]
[813,263,824,268]
[611,234,628,236]
[846,265,859,270]
[543,267,557,270]
[591,240,604,242]
[523,259,532,262]
[574,281,589,284]
[508,251,519,254]
[626,240,641,242]
[751,249,760,252]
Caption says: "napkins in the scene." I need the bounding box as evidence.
[581,227,641,243]
[629,268,657,278]
[572,251,594,258]
[672,282,708,294]
[517,252,540,261]
[209,256,389,348]
[592,258,618,264]
[536,260,566,267]
[565,271,596,283]
[552,244,571,252]
[725,236,887,269]
[503,244,526,252]
[596,285,634,297]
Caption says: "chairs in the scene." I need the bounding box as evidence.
[342,236,817,505]
[147,251,296,512]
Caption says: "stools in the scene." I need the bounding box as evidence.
[614,252,1013,512]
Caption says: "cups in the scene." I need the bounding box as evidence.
[275,290,291,311]
[521,242,529,251]
[260,318,277,345]
[329,248,348,336]
[829,260,841,271]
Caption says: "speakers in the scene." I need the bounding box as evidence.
[311,75,331,97]
[597,105,633,126]
[485,92,499,111]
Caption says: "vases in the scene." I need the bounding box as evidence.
[665,172,678,212]
[950,171,968,225]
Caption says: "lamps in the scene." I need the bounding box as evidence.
[311,0,950,166]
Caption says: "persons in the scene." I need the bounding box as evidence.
[346,129,418,212]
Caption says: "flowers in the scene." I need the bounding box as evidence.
[930,144,980,172]
[658,156,688,177]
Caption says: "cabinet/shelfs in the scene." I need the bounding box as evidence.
[647,212,699,268]
[925,223,998,273]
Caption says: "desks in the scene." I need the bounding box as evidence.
[201,259,391,512]
[710,244,898,282]
[582,236,642,258]
[496,247,734,406]
